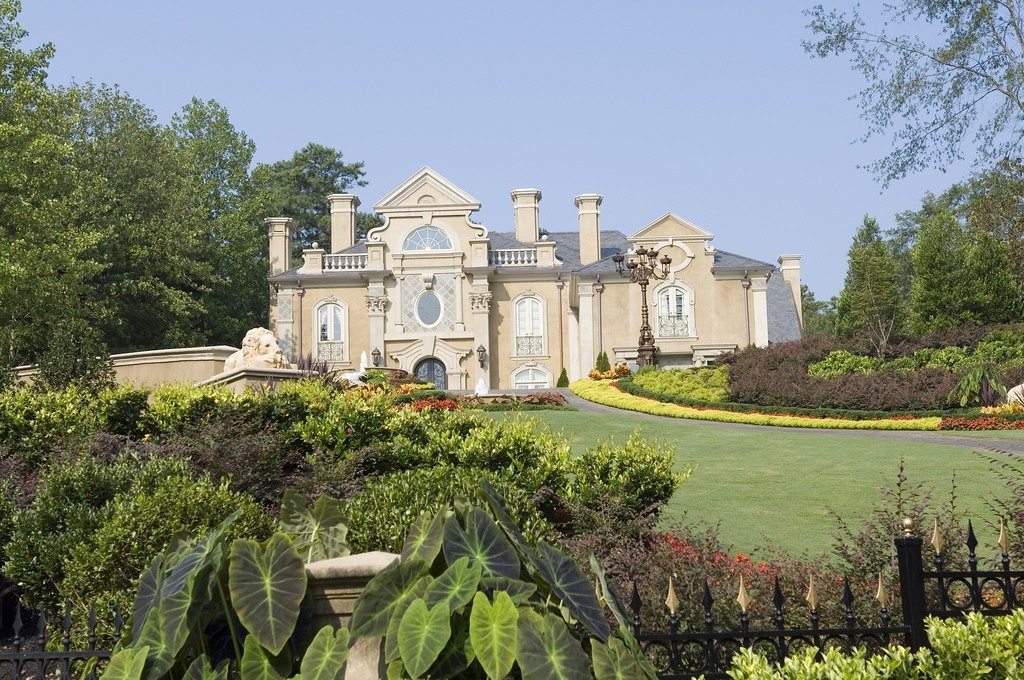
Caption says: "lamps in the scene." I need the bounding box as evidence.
[476,344,486,368]
[371,347,381,367]
